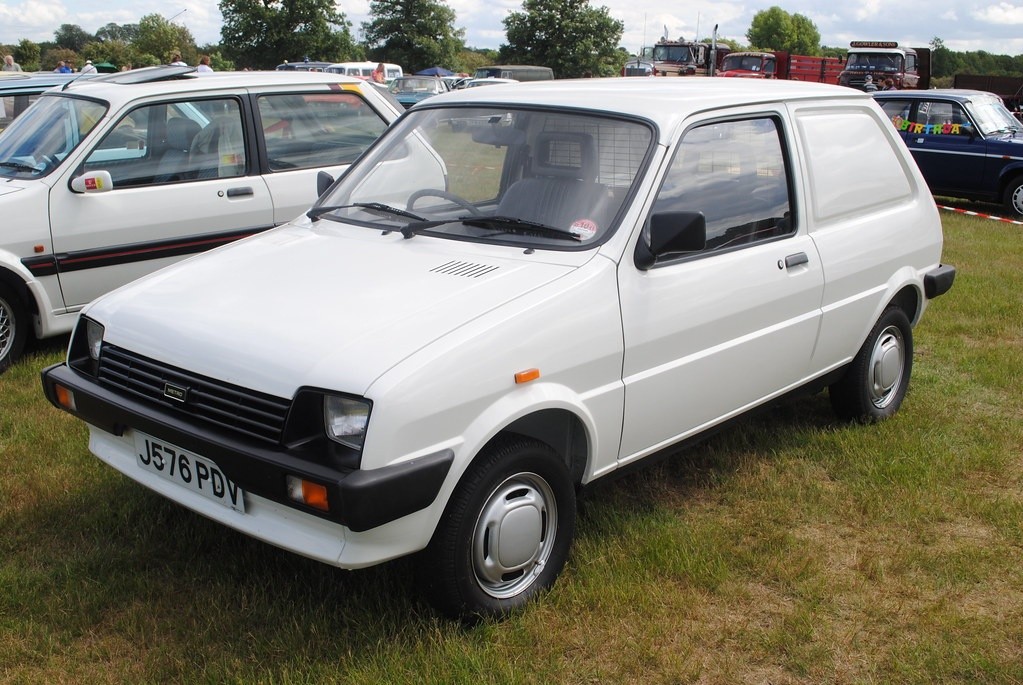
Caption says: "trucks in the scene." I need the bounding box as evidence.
[840,39,931,93]
[640,24,730,77]
[714,49,846,84]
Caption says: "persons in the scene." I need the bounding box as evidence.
[862,75,878,91]
[170,50,186,65]
[194,56,213,72]
[241,65,262,72]
[373,63,386,85]
[1,55,23,73]
[431,72,442,79]
[877,76,885,90]
[117,62,141,72]
[81,60,98,74]
[728,58,773,74]
[583,71,593,79]
[884,78,896,90]
[55,56,77,73]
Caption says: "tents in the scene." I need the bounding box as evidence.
[79,60,117,73]
[414,65,453,77]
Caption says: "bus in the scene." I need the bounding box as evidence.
[474,66,554,82]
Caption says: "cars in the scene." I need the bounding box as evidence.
[34,72,959,612]
[450,79,520,131]
[439,76,472,93]
[0,71,210,171]
[385,76,448,112]
[0,66,448,364]
[868,86,1023,214]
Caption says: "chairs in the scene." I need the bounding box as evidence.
[146,117,236,186]
[494,130,615,242]
[902,108,972,136]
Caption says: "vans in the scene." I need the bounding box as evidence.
[325,61,403,90]
[277,59,333,74]
